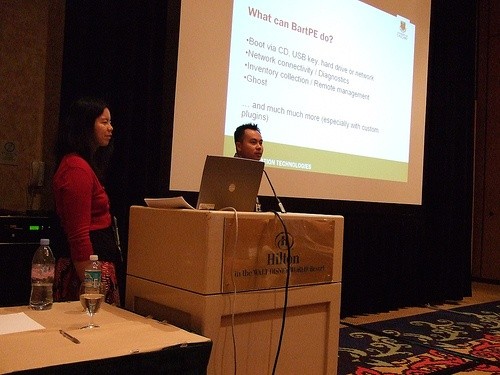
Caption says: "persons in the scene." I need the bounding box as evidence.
[52,97,122,307]
[234,123,263,160]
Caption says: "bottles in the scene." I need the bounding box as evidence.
[30,239,56,310]
[84,254,100,295]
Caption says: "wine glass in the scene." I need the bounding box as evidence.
[79,282,105,329]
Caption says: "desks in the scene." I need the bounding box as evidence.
[0,301,212,375]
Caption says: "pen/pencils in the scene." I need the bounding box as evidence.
[59,329,80,344]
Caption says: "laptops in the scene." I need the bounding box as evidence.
[196,154,265,212]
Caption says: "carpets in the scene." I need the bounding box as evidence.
[337,299,500,375]
